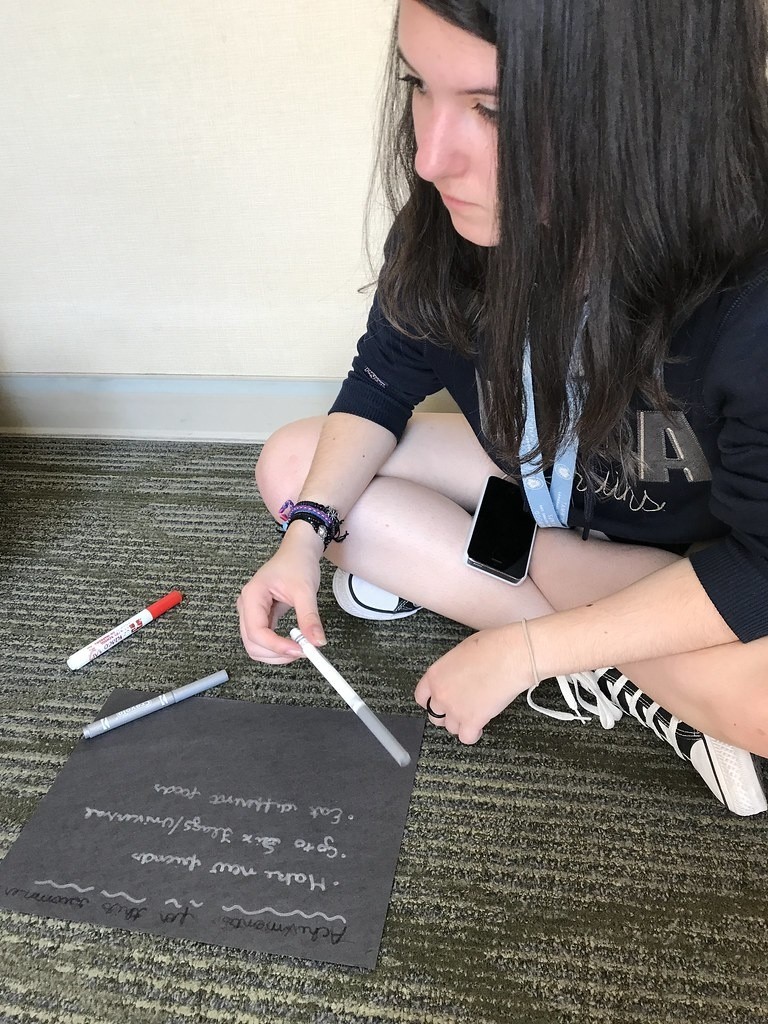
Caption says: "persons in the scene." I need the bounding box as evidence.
[234,0,768,818]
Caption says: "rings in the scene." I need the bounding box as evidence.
[427,697,445,718]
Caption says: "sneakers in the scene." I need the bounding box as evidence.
[332,567,424,620]
[527,666,767,816]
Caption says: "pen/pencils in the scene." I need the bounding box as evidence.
[289,628,411,767]
[67,591,183,671]
[82,670,229,739]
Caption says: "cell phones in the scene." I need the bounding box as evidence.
[465,475,538,586]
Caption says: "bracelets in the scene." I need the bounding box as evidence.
[521,618,539,688]
[279,500,349,553]
